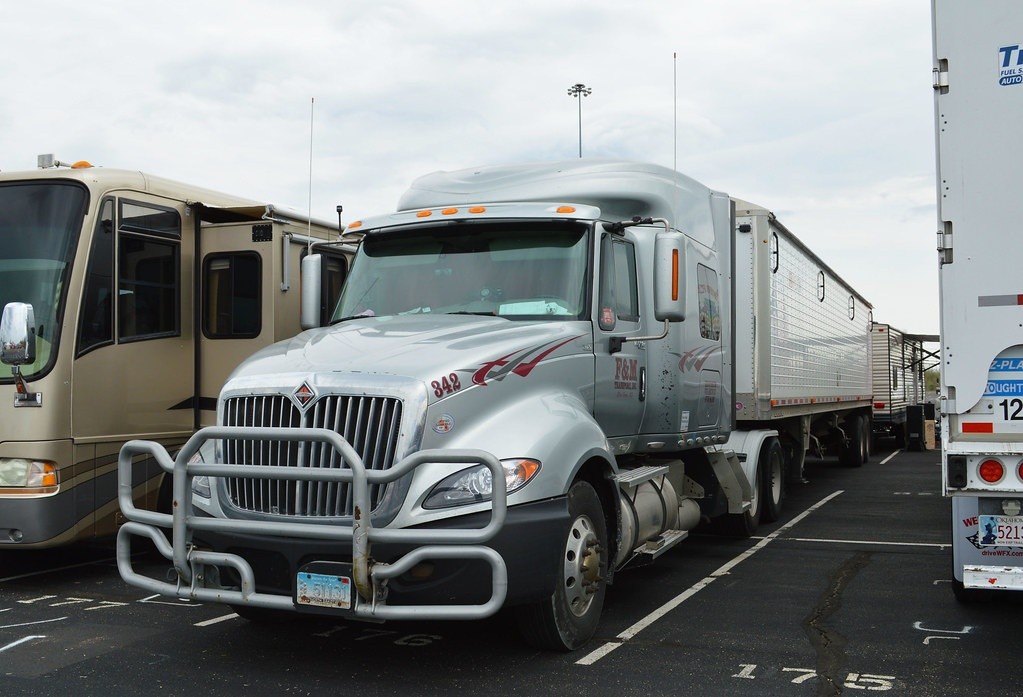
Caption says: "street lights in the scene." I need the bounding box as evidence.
[567,83,592,159]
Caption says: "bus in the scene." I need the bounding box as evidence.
[0,155,364,567]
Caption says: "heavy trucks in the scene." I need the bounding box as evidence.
[117,160,924,652]
[930,0,1023,603]
